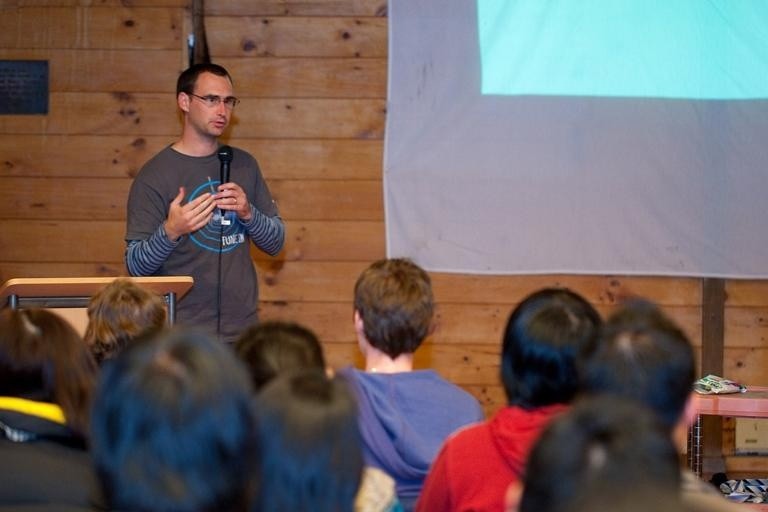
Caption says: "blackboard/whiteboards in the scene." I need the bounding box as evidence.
[0,60,49,115]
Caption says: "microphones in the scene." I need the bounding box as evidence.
[216,143,235,217]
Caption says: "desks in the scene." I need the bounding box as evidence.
[686,390,768,475]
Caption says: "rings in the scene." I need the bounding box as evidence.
[233,198,236,204]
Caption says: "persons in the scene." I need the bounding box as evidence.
[89,319,255,512]
[124,61,285,348]
[0,308,107,512]
[504,392,681,512]
[235,320,406,512]
[251,366,363,512]
[577,302,701,439]
[332,256,484,512]
[414,286,604,512]
[83,278,167,364]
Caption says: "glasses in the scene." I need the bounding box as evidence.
[185,91,240,107]
